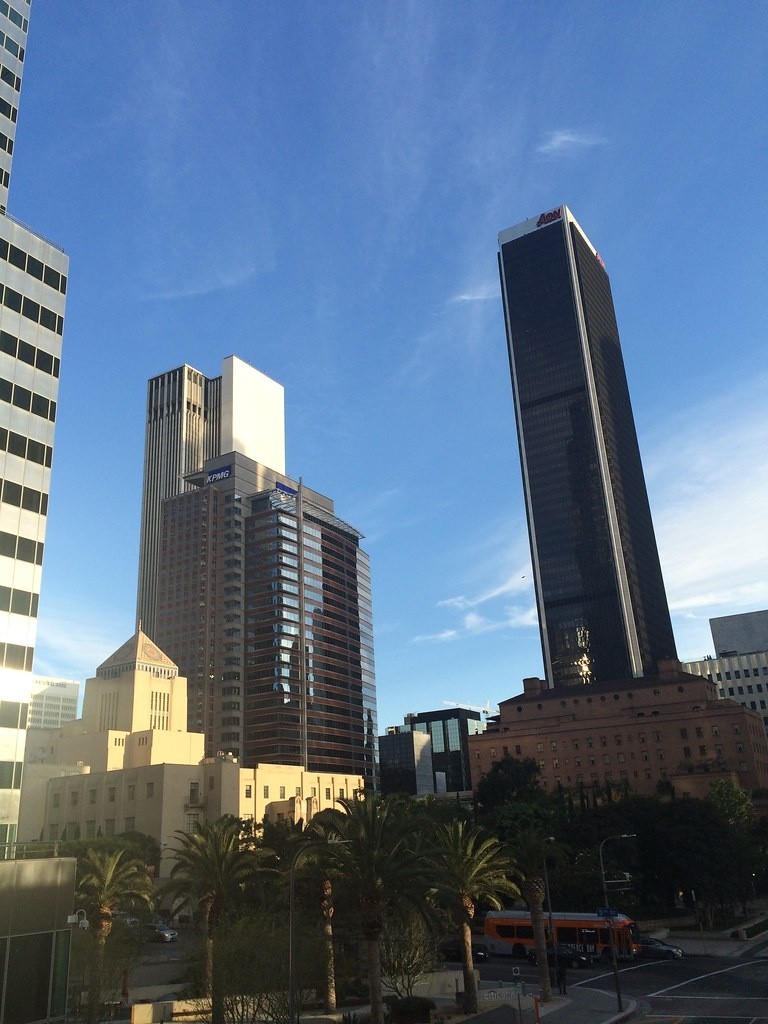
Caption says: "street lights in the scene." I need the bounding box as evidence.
[541,837,557,988]
[600,835,636,1013]
[288,839,357,1024]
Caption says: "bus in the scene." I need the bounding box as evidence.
[483,908,641,964]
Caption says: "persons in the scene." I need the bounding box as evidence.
[557,967,567,995]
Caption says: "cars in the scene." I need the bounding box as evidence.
[132,924,177,943]
[110,911,139,927]
[637,938,687,960]
[67,983,96,1008]
[528,947,594,967]
[438,940,489,964]
